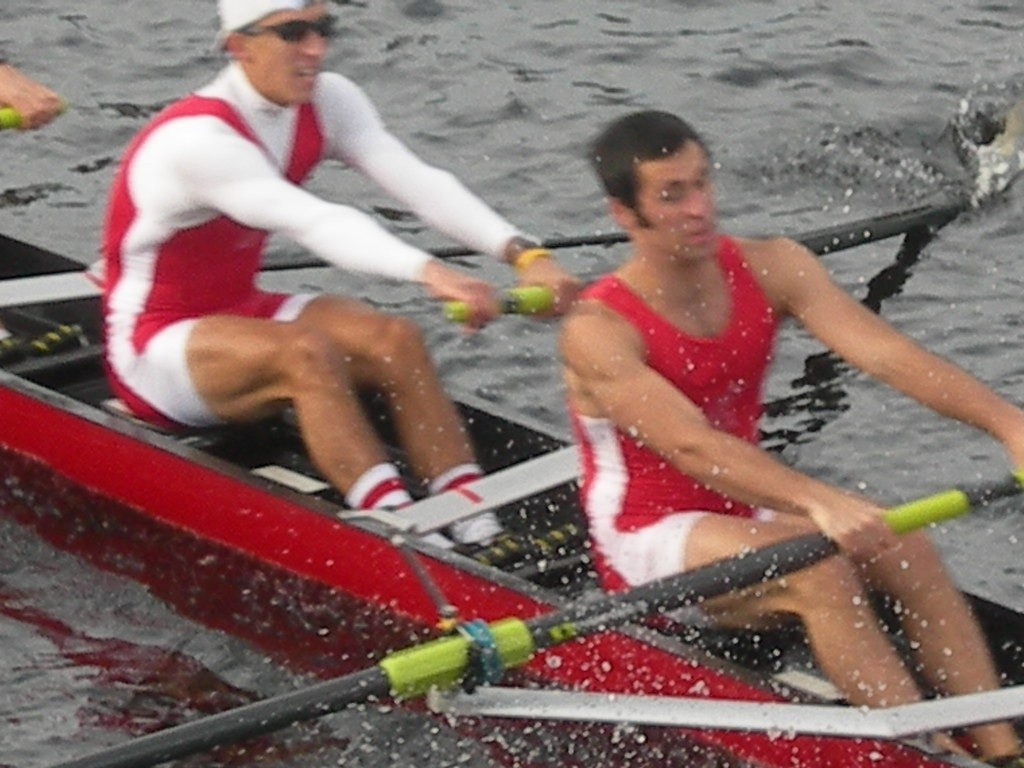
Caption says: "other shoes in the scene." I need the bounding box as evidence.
[450,532,530,569]
[525,524,584,563]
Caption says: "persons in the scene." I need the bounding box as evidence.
[1,62,62,133]
[100,0,576,548]
[556,112,1024,768]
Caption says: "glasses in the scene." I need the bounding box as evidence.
[242,14,337,41]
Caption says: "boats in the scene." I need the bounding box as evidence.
[0,227,1024,767]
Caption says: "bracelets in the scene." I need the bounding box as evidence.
[519,249,547,268]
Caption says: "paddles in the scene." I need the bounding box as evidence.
[438,198,967,328]
[256,231,632,271]
[0,97,70,131]
[50,468,1024,768]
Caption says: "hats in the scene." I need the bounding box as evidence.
[212,0,334,57]
